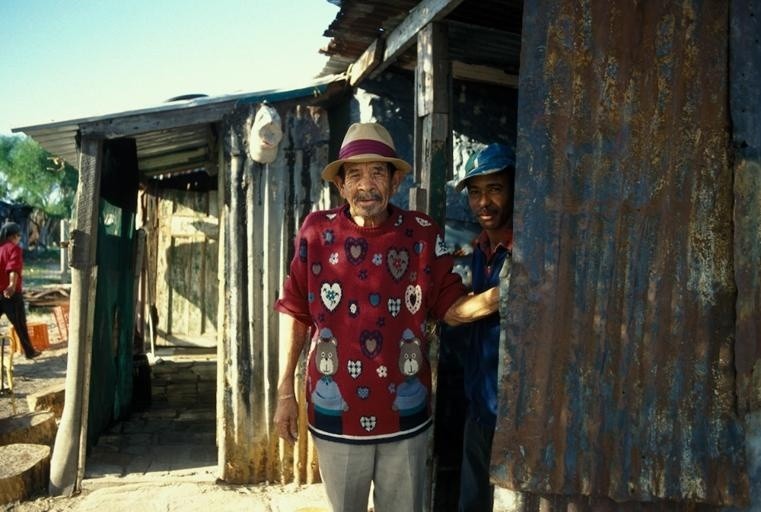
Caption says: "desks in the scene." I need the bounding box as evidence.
[16,298,70,355]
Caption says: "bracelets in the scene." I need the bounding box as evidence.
[277,394,299,401]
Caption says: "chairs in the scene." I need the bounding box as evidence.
[0,336,16,417]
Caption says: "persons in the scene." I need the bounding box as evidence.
[0,220,43,361]
[432,138,516,511]
[274,123,503,512]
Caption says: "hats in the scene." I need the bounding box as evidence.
[455,142,515,194]
[320,122,413,183]
[248,104,283,166]
